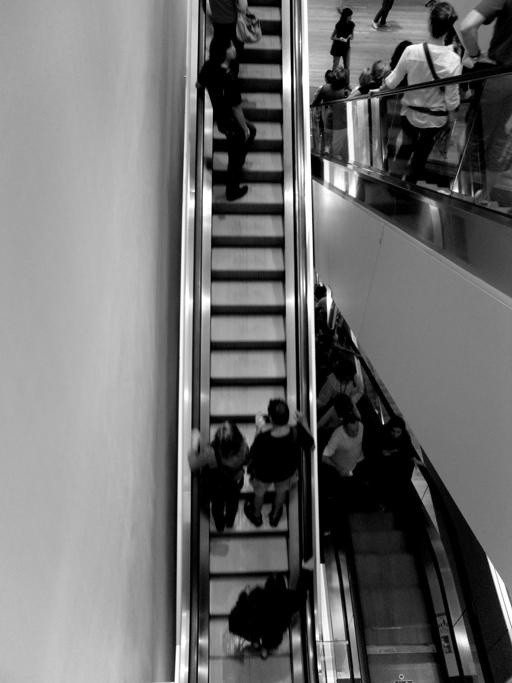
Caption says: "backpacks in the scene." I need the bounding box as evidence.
[209,463,239,498]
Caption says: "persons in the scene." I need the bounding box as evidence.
[313,41,413,169]
[237,558,313,660]
[243,399,314,526]
[191,423,250,532]
[370,1,462,184]
[330,8,356,69]
[440,0,511,199]
[316,284,413,534]
[195,32,258,202]
[200,0,251,61]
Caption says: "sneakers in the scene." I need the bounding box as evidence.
[244,499,282,527]
[227,185,248,200]
[371,19,389,28]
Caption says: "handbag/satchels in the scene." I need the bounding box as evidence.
[235,12,262,43]
